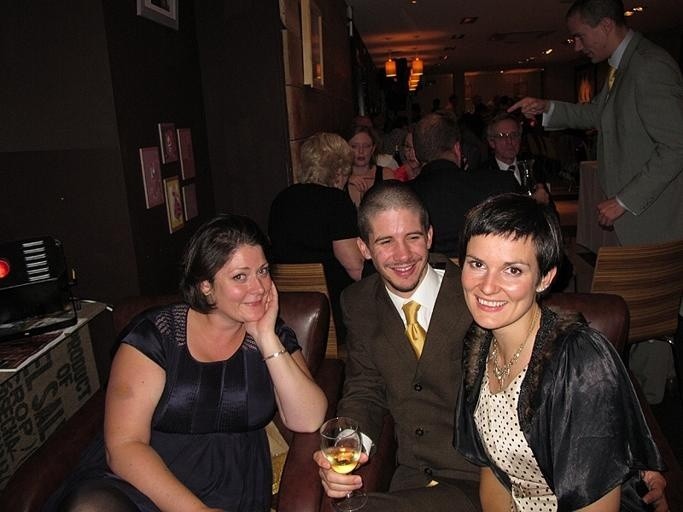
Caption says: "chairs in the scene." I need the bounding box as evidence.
[590,238,683,406]
[268,262,348,364]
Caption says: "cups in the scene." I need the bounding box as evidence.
[517,158,535,192]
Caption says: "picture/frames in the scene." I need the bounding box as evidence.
[139,146,164,209]
[182,182,199,221]
[158,122,178,165]
[301,1,325,94]
[136,1,179,32]
[177,127,196,180]
[163,175,185,234]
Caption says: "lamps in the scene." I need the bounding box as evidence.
[385,48,396,77]
[411,35,423,76]
[409,87,416,91]
[409,81,418,88]
[410,75,419,82]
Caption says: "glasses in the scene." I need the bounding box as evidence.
[348,142,378,153]
[486,130,524,141]
[565,18,610,45]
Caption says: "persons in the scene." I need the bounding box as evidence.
[313,183,669,512]
[269,131,365,281]
[343,129,391,206]
[442,94,464,113]
[470,95,489,140]
[393,129,423,182]
[508,2,683,250]
[456,194,665,512]
[472,113,561,221]
[408,114,487,253]
[70,211,328,512]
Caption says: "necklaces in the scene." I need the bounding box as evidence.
[487,304,541,391]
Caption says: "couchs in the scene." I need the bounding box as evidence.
[318,290,683,511]
[1,292,345,512]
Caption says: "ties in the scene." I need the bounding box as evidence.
[400,301,428,357]
[508,163,517,180]
[605,65,614,90]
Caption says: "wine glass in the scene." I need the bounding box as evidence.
[320,417,368,511]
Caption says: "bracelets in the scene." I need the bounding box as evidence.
[260,349,290,362]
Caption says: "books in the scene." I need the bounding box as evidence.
[0,331,64,372]
